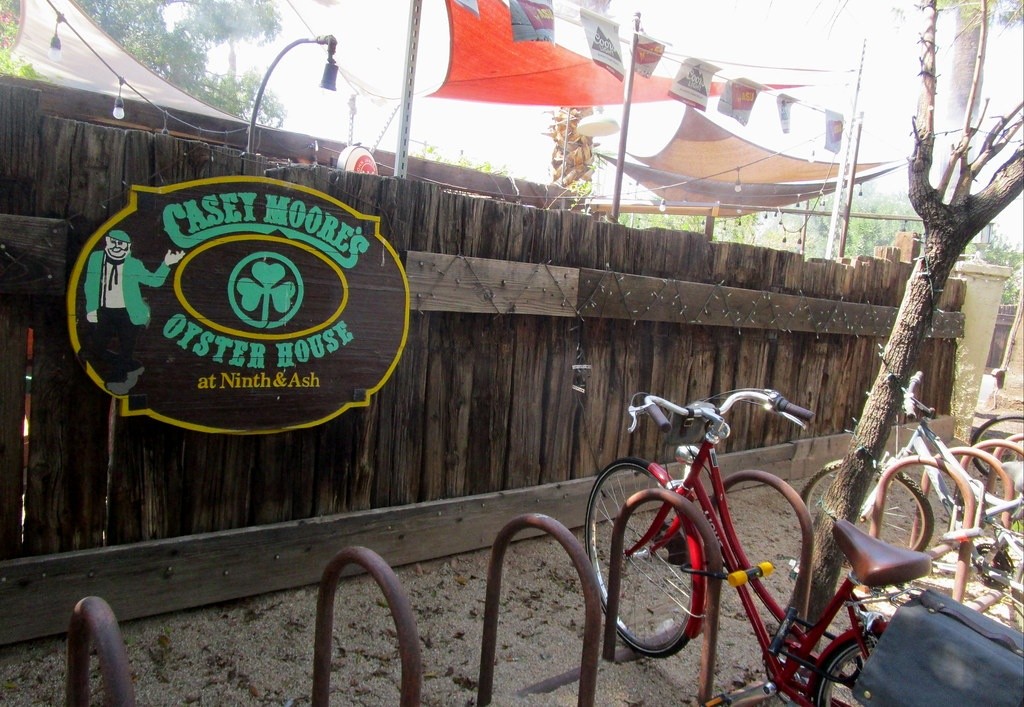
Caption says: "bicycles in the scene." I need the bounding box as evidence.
[796,369,1023,624]
[585,389,933,707]
